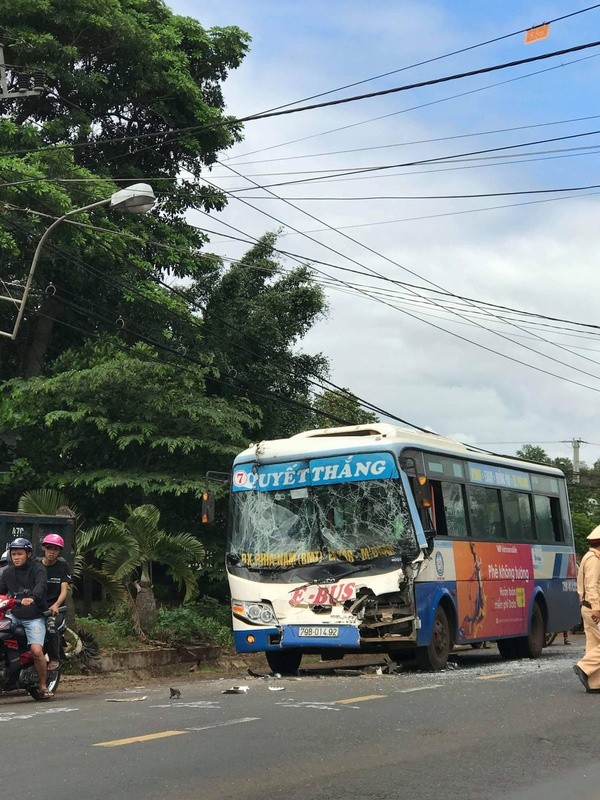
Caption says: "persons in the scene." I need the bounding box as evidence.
[35,534,72,670]
[0,538,55,699]
[572,526,600,692]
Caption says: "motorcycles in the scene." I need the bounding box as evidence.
[0,589,66,702]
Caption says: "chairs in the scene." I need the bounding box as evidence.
[491,521,504,537]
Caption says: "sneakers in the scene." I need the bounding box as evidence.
[573,665,590,689]
[586,688,600,694]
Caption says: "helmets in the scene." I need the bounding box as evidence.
[41,534,64,548]
[9,538,33,552]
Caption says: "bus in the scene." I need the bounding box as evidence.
[204,421,583,680]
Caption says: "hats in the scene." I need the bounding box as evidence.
[587,525,600,544]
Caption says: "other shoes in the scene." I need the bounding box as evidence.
[47,659,59,670]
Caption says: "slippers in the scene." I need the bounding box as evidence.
[40,689,54,697]
